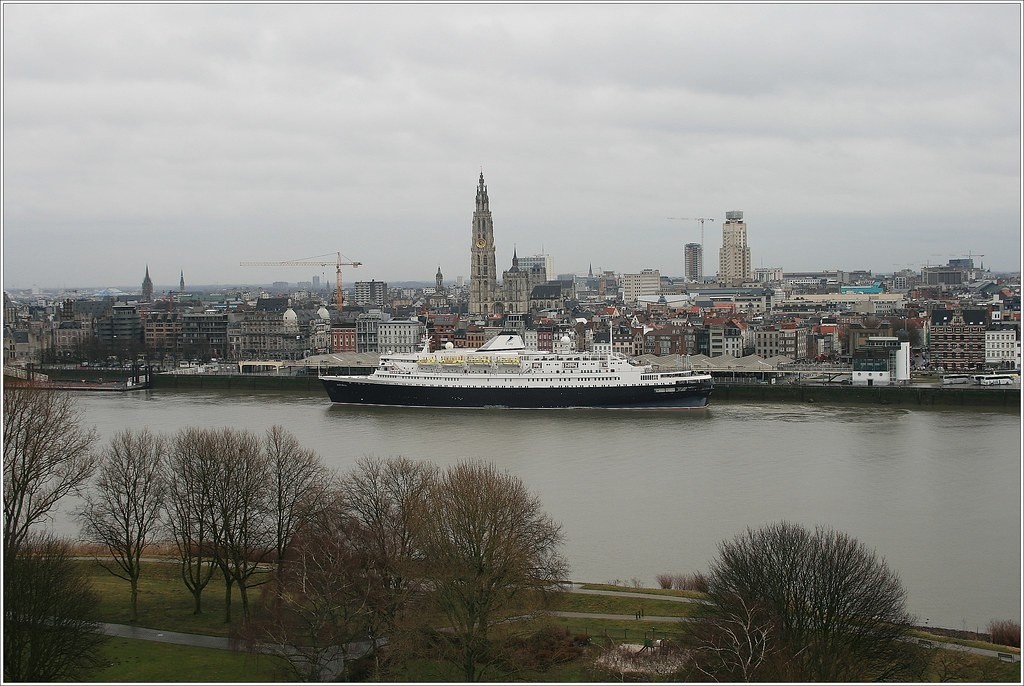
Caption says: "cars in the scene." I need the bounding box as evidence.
[81,359,162,368]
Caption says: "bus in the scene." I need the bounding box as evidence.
[939,375,969,384]
[980,375,1013,386]
[970,375,981,384]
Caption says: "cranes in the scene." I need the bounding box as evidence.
[667,217,714,275]
[239,251,362,314]
[962,250,984,259]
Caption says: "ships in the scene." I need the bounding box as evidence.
[317,322,716,410]
